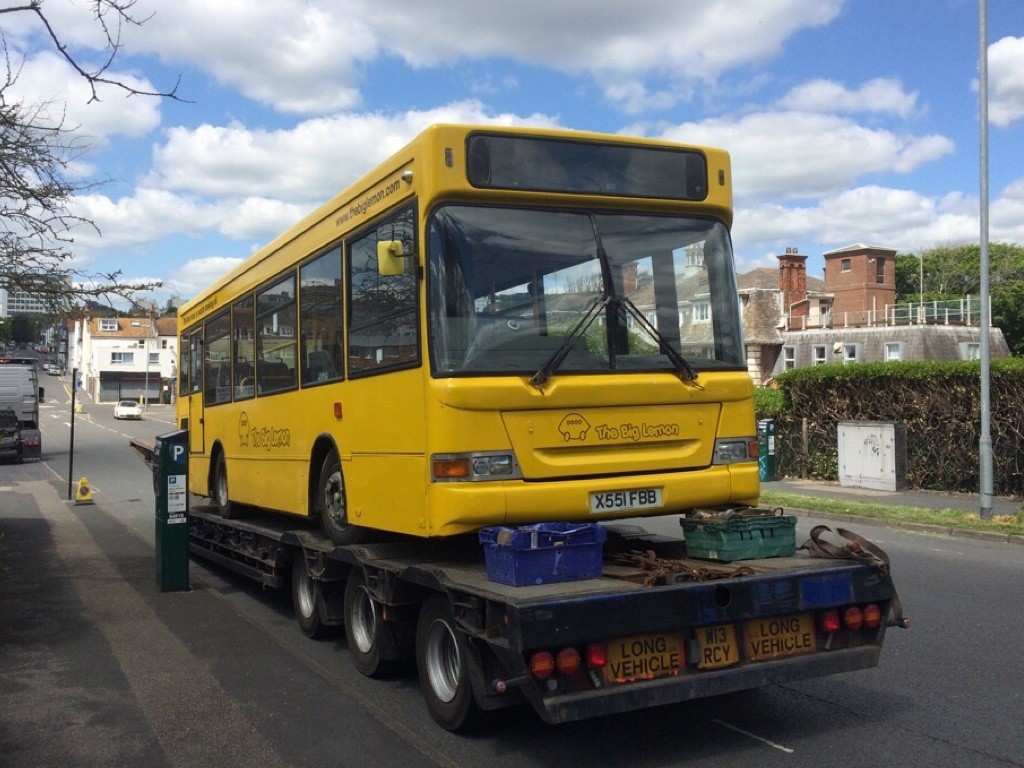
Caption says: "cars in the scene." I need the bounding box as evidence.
[114,401,142,420]
[49,367,61,375]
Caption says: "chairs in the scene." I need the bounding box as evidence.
[204,349,335,402]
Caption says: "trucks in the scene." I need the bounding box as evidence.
[0,357,45,463]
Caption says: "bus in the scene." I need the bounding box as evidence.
[176,123,761,543]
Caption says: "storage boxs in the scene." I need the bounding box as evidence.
[479,522,609,586]
[681,514,797,561]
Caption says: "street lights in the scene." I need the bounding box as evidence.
[132,323,150,413]
